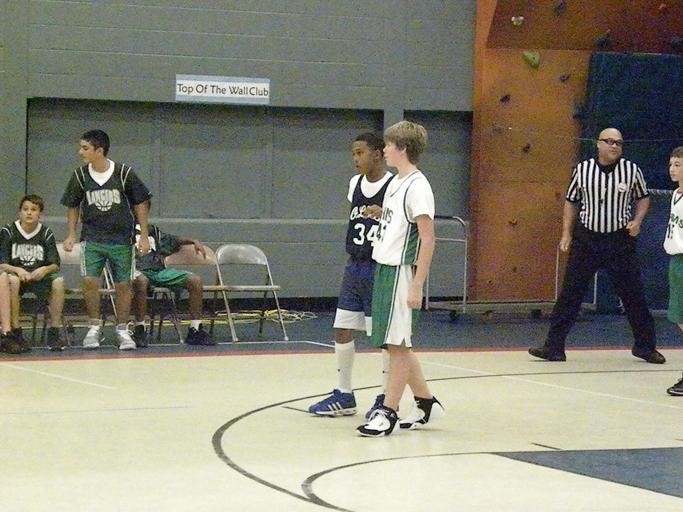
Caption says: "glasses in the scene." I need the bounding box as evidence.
[590,137,624,146]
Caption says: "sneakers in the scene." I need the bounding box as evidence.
[308,386,358,421]
[364,394,386,418]
[130,321,150,353]
[397,396,454,436]
[628,339,667,367]
[79,321,107,349]
[43,320,67,352]
[12,325,32,353]
[0,322,29,354]
[526,341,568,367]
[113,320,137,351]
[354,406,400,440]
[662,376,681,397]
[183,323,222,347]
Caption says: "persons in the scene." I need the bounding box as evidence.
[307,131,395,422]
[354,121,446,440]
[59,128,153,352]
[526,126,669,364]
[133,220,217,348]
[0,271,21,354]
[0,195,67,354]
[662,143,683,396]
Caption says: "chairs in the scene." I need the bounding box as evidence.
[156,243,236,343]
[211,243,289,343]
[101,259,182,346]
[41,243,118,347]
[19,292,50,348]
